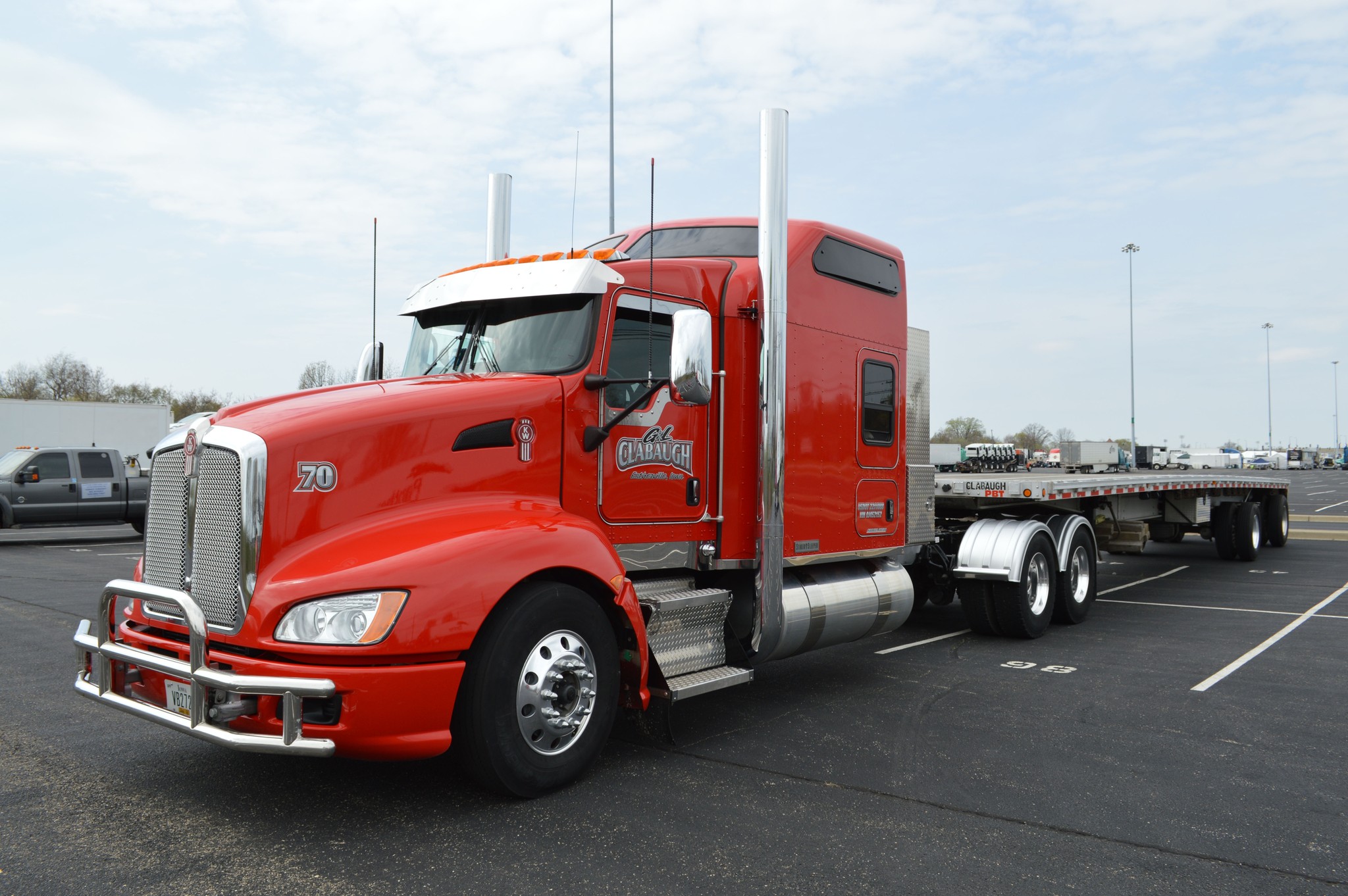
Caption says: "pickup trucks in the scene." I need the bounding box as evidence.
[0,446,154,534]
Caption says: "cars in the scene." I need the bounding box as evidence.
[1323,455,1348,470]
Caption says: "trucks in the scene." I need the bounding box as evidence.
[63,192,1288,799]
[1151,450,1287,473]
[1058,441,1129,476]
[927,442,1020,474]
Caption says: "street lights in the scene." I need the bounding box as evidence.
[1261,323,1275,465]
[1332,360,1340,451]
[1123,243,1140,465]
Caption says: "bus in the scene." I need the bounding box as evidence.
[1287,449,1314,469]
